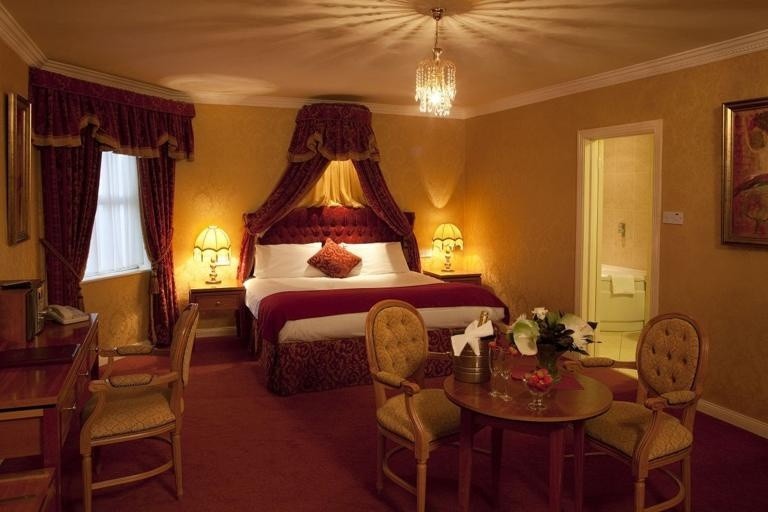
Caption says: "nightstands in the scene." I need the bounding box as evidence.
[189,280,249,349]
[423,267,482,285]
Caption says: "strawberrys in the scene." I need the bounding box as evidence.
[525,367,552,391]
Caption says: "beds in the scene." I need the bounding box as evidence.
[237,209,510,397]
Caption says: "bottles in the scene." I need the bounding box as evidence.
[477,311,495,342]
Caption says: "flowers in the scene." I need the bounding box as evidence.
[501,307,601,383]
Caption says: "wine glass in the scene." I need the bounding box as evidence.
[501,350,513,402]
[526,382,552,412]
[487,346,502,400]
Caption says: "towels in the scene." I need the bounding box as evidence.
[608,273,635,295]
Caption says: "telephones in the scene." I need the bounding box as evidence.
[47,304,89,324]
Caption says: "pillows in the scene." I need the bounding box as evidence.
[253,242,327,278]
[308,237,361,278]
[342,242,410,277]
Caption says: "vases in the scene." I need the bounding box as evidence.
[536,344,563,381]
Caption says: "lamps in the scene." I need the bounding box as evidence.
[413,1,473,117]
[432,222,463,272]
[195,225,232,284]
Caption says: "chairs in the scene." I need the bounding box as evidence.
[78,302,200,511]
[365,299,503,512]
[568,312,709,511]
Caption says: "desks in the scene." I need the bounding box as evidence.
[0,470,60,512]
[0,309,100,512]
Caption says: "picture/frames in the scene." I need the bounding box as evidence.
[6,91,31,246]
[722,95,767,249]
